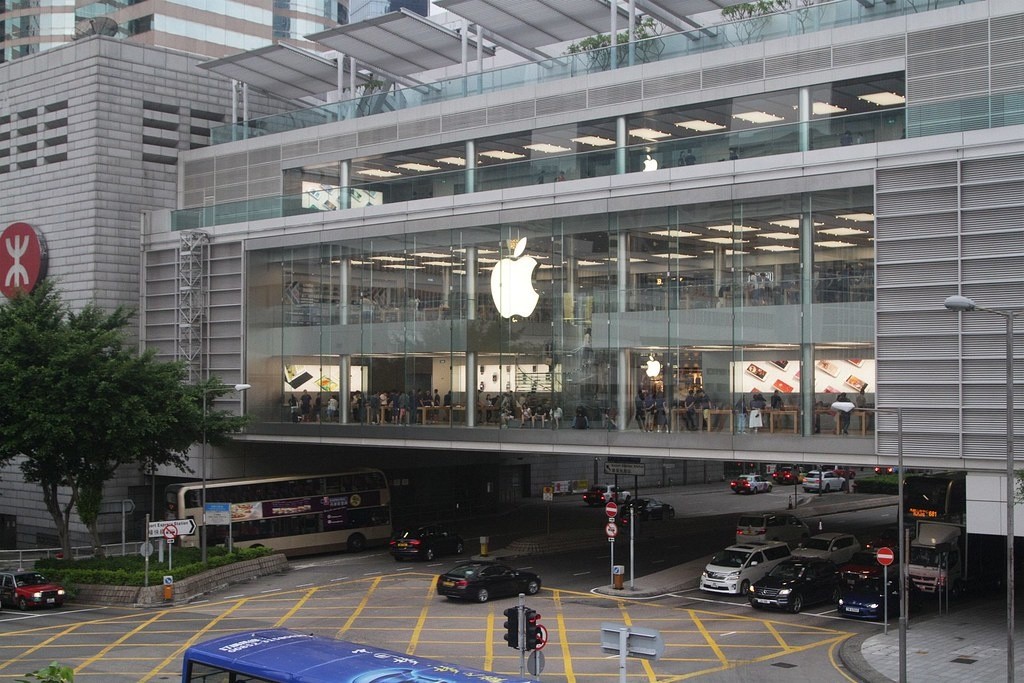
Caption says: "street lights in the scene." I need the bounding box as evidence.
[202,383,252,565]
[944,295,1024,683]
[832,402,907,683]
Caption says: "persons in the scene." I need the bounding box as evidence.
[352,389,440,426]
[856,390,866,412]
[536,170,545,184]
[444,391,451,406]
[486,390,514,428]
[839,130,865,147]
[837,393,851,434]
[747,272,771,306]
[735,394,748,434]
[557,171,565,182]
[770,390,784,428]
[678,149,697,167]
[635,390,672,433]
[519,394,526,405]
[290,389,338,422]
[729,147,740,160]
[571,406,590,429]
[359,295,378,323]
[684,389,712,431]
[750,393,766,410]
[520,403,563,429]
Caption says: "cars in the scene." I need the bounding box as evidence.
[0,568,66,611]
[437,559,542,603]
[731,466,855,494]
[620,497,674,520]
[390,522,465,562]
[746,533,897,620]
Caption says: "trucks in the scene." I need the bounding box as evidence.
[907,521,973,600]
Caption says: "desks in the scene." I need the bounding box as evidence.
[381,307,447,320]
[670,406,872,435]
[291,404,465,423]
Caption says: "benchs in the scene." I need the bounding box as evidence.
[509,418,606,428]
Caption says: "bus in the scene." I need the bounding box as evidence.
[182,628,541,683]
[163,468,393,557]
[897,469,967,538]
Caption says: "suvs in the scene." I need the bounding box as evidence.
[583,483,631,506]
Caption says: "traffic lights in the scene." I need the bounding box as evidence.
[502,607,518,647]
[620,506,630,527]
[525,610,543,649]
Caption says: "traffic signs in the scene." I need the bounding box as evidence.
[149,520,197,538]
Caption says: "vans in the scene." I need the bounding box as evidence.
[699,511,812,595]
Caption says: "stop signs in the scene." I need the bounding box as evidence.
[877,547,894,565]
[606,502,618,517]
